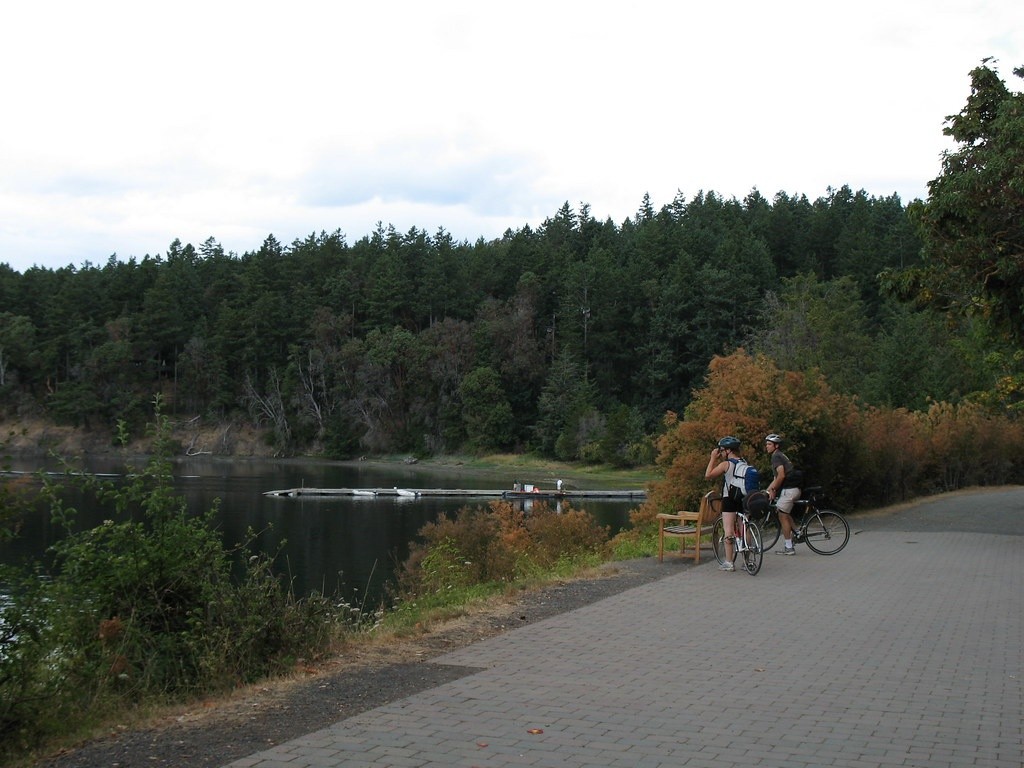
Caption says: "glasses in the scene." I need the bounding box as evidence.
[720,448,728,452]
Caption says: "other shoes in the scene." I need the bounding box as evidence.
[719,561,734,571]
[775,546,795,556]
[741,561,755,570]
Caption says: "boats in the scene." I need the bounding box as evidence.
[352,490,379,497]
[396,489,422,498]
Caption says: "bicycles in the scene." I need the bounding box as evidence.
[746,490,850,556]
[709,497,764,575]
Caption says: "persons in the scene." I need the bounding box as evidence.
[557,478,563,490]
[513,477,519,490]
[764,434,802,556]
[704,435,756,572]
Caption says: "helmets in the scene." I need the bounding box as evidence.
[766,434,783,443]
[718,436,741,447]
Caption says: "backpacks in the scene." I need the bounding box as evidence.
[724,458,759,501]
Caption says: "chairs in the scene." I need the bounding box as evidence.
[656,491,722,564]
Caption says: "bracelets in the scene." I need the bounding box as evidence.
[770,486,777,492]
